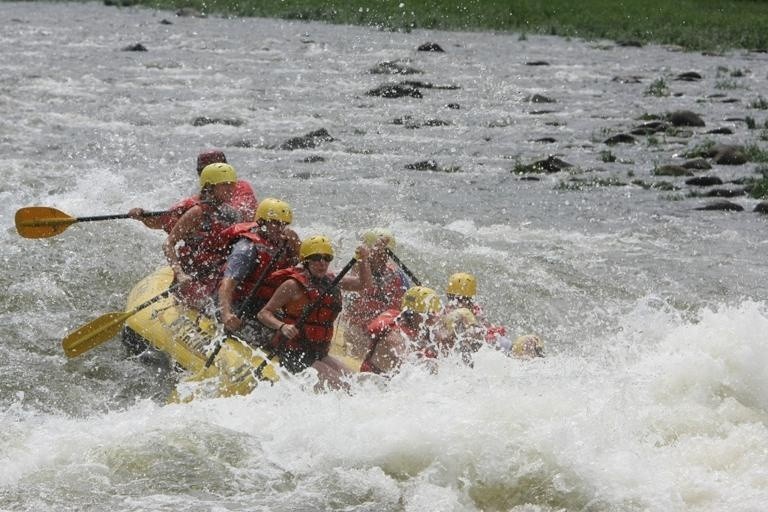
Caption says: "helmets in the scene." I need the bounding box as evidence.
[200,163,236,191]
[513,336,543,355]
[254,198,292,224]
[362,228,395,250]
[447,273,476,297]
[198,151,227,173]
[299,236,334,261]
[446,307,474,334]
[400,287,441,313]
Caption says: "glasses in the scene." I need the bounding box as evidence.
[271,219,287,230]
[309,254,333,262]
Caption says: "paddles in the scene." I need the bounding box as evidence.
[217,254,360,397]
[15,200,219,238]
[167,239,288,404]
[63,259,226,358]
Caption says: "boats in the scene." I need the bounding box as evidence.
[122,260,545,408]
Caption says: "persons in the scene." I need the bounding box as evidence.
[126,148,259,247]
[160,161,255,317]
[256,236,373,395]
[212,198,300,343]
[354,228,409,310]
[361,270,542,382]
